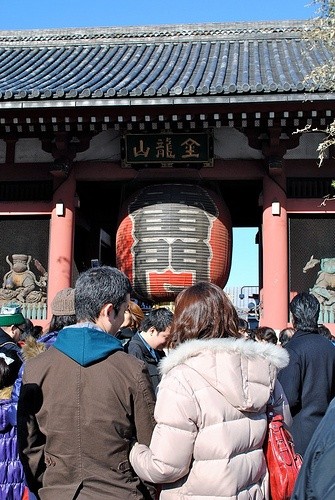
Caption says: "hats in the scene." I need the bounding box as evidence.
[0,302,25,326]
[50,288,76,316]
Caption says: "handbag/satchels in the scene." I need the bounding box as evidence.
[266,404,303,500]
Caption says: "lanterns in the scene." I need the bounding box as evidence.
[116,177,234,311]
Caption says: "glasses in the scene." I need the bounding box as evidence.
[15,326,25,334]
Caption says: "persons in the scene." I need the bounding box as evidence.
[129,284,303,500]
[235,295,335,500]
[0,267,175,500]
